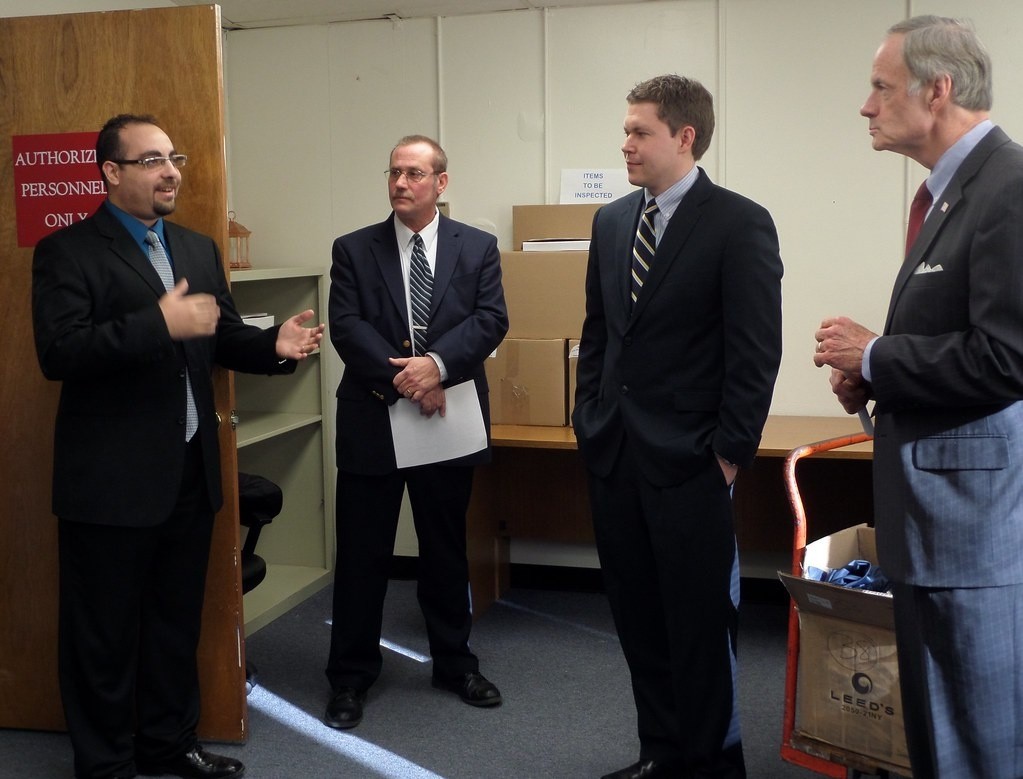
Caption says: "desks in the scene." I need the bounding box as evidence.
[465,415,874,617]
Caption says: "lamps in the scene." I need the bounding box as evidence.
[228,209,252,270]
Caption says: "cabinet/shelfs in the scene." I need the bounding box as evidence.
[228,267,335,636]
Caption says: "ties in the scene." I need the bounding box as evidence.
[906,178,932,261]
[145,230,198,442]
[631,198,661,312]
[410,232,433,357]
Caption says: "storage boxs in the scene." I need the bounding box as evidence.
[497,340,566,427]
[484,348,496,425]
[498,203,607,339]
[568,339,580,427]
[776,523,914,779]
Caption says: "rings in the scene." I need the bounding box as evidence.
[407,390,413,395]
[818,342,822,353]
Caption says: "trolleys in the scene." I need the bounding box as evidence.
[781,430,913,779]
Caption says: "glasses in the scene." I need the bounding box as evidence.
[113,154,189,170]
[384,169,440,182]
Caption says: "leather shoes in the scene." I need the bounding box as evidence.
[601,756,656,779]
[135,746,245,779]
[432,670,501,706]
[325,689,368,728]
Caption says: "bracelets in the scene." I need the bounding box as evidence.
[721,458,735,468]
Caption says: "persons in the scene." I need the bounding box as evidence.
[814,15,1023,779]
[31,112,325,779]
[327,133,510,733]
[569,75,785,779]
